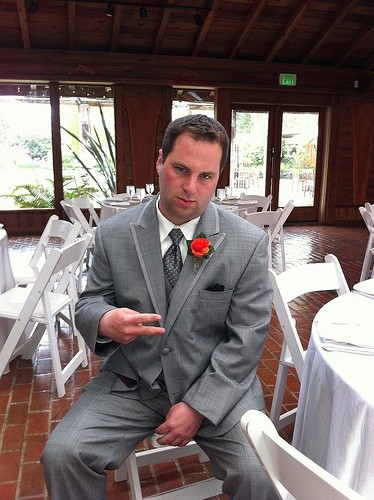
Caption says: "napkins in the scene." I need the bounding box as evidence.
[316,321,374,348]
[352,279,374,299]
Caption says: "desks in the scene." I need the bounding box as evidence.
[0,223,15,294]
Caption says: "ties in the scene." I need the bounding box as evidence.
[163,229,185,288]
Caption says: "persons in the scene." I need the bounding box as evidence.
[40,115,274,500]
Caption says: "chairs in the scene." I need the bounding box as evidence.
[113,193,373,499]
[0,196,99,398]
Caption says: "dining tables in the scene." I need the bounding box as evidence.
[101,192,259,222]
[291,292,374,500]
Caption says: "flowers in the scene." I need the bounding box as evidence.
[186,231,215,259]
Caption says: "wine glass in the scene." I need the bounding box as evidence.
[136,188,145,204]
[145,184,154,196]
[126,186,135,201]
[225,186,232,201]
[217,189,225,205]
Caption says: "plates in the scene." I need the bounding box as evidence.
[352,278,374,296]
[234,200,258,205]
[104,196,125,202]
